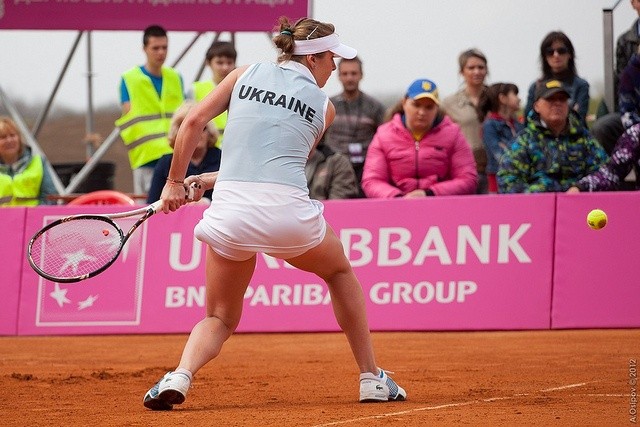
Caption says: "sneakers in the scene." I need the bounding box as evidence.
[143,370,191,410]
[359,367,407,402]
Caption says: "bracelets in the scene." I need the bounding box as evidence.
[164,177,186,187]
[423,188,437,198]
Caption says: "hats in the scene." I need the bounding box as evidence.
[406,79,440,106]
[534,79,570,101]
[282,32,357,60]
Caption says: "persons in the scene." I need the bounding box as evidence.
[477,83,524,196]
[441,49,491,194]
[0,116,59,206]
[305,131,361,198]
[590,0,640,155]
[147,105,221,204]
[618,34,640,129]
[360,79,479,198]
[496,80,610,196]
[566,123,640,193]
[143,16,408,411]
[114,27,185,197]
[323,57,387,198]
[524,33,589,132]
[186,42,238,150]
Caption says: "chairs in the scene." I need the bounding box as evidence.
[68,190,135,204]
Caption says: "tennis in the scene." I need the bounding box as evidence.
[587,209,607,230]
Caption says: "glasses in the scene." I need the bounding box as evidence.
[544,48,569,54]
